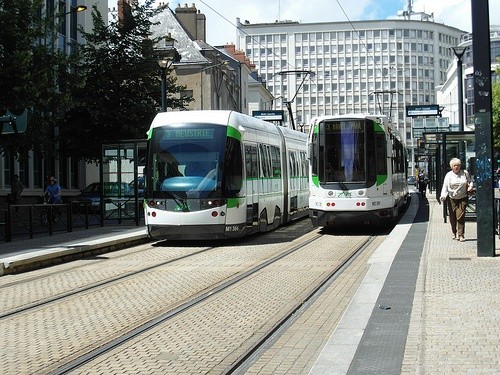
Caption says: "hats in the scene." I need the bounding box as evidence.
[49,177,57,181]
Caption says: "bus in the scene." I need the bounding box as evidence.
[306,90,411,230]
[143,70,316,241]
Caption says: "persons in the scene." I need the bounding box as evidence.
[418,170,427,200]
[10,175,23,205]
[44,178,61,216]
[440,158,474,241]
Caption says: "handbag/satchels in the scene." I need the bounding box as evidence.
[465,171,473,196]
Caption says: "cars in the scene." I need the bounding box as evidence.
[69,175,144,215]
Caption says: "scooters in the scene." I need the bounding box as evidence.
[39,196,65,227]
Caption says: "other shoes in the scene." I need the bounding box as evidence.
[460,237,465,242]
[452,233,456,239]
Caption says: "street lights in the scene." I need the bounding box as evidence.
[449,46,470,170]
